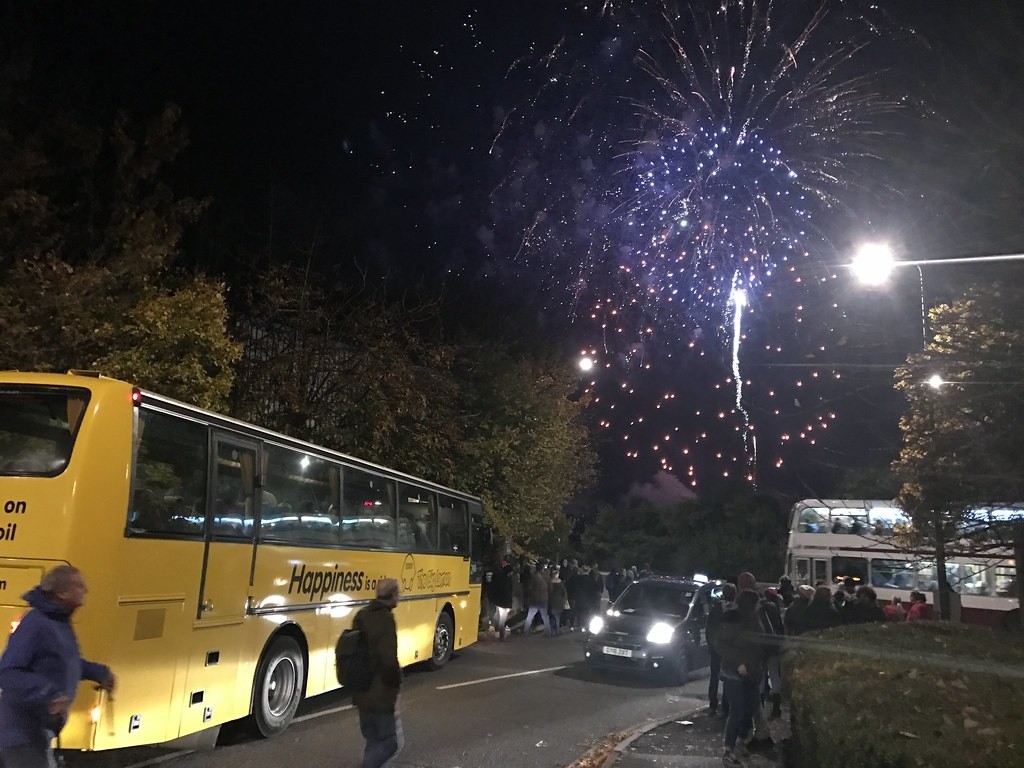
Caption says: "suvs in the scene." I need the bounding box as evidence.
[582,574,733,687]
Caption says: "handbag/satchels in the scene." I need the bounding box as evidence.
[564,600,570,610]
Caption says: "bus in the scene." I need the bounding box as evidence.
[0,366,495,757]
[782,498,1024,633]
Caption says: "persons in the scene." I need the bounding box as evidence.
[0,564,116,768]
[350,578,405,768]
[471,549,1018,768]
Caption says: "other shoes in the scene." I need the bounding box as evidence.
[485,622,590,642]
[708,691,781,767]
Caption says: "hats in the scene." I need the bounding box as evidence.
[766,588,777,600]
[738,572,758,593]
[798,584,815,598]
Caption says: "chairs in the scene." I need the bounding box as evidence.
[124,490,430,550]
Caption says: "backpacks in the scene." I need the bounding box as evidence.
[335,608,386,690]
